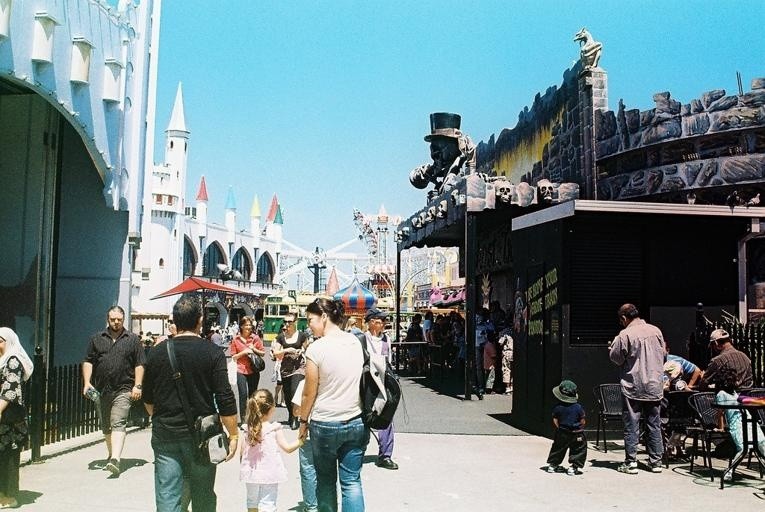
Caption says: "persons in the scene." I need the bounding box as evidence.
[345,319,359,335]
[291,342,318,511]
[140,324,177,348]
[238,388,309,511]
[142,296,239,511]
[547,378,588,474]
[231,316,265,431]
[607,303,764,482]
[358,307,401,471]
[205,319,264,353]
[406,300,513,399]
[0,326,33,510]
[81,303,147,478]
[297,297,366,511]
[269,313,313,428]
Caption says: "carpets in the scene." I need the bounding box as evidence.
[230,351,535,436]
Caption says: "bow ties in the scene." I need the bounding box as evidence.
[617,461,639,474]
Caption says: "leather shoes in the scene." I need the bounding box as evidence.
[290,416,298,430]
[378,459,398,469]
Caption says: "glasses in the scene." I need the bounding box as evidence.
[373,316,386,322]
[284,321,293,323]
[314,297,327,317]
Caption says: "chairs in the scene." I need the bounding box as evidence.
[591,382,765,497]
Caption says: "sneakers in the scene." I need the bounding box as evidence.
[645,461,663,473]
[0,497,18,510]
[106,459,120,475]
[568,466,583,476]
[103,456,111,470]
[548,464,565,473]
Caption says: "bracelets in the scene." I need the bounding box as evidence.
[227,433,239,442]
[299,416,309,425]
[134,383,141,390]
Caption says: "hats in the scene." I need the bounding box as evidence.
[364,308,391,322]
[553,380,579,404]
[708,329,730,349]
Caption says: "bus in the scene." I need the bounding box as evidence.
[384,312,425,332]
[263,291,335,347]
[419,308,454,322]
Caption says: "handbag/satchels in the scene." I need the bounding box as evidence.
[192,412,229,467]
[354,332,401,430]
[250,352,265,373]
